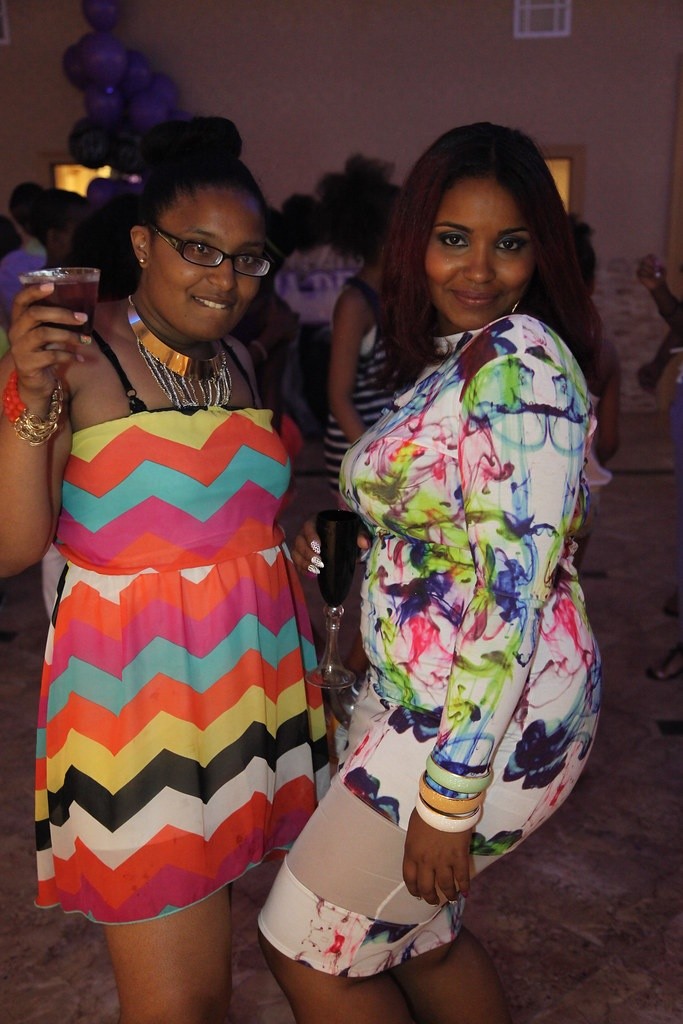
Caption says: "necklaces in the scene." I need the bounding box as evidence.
[128,294,233,411]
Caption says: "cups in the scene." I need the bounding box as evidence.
[19,262,99,349]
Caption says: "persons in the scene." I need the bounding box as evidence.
[0,151,621,572]
[260,120,600,1024]
[637,254,683,678]
[0,114,337,1024]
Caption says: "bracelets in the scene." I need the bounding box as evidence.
[3,370,64,446]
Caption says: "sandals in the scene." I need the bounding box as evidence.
[645,640,683,680]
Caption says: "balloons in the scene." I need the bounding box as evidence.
[64,0,191,212]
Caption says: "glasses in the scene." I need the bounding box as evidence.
[143,219,275,277]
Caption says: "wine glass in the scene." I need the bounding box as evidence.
[306,508,370,690]
